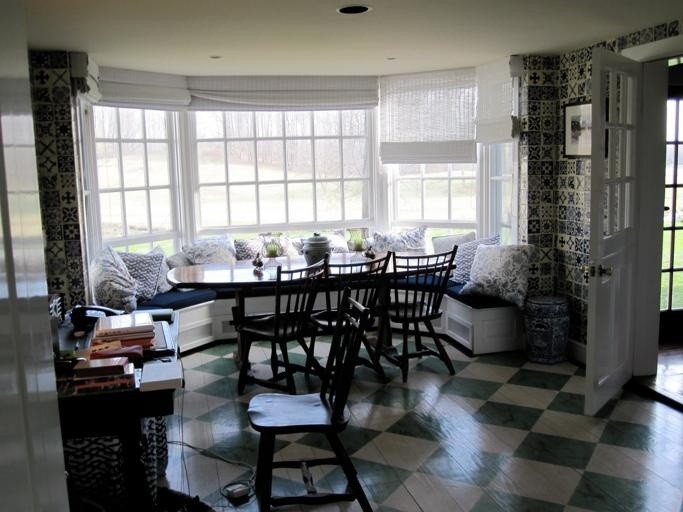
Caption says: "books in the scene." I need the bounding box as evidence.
[53,309,156,400]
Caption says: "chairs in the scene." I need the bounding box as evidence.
[247,288,374,512]
[238,256,325,397]
[305,251,390,389]
[375,245,457,383]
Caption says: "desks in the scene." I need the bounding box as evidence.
[56,310,183,512]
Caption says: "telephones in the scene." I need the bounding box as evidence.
[70,304,125,326]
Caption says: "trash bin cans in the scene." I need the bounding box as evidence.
[524,295,570,365]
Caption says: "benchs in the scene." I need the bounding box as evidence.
[140,273,522,358]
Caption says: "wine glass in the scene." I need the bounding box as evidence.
[345,227,370,262]
[261,231,284,267]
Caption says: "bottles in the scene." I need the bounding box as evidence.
[301,235,330,269]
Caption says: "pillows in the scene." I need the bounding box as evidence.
[370,224,429,252]
[458,241,535,309]
[181,232,236,266]
[88,243,139,311]
[166,251,191,271]
[118,248,164,300]
[234,237,296,257]
[292,227,350,254]
[147,243,173,295]
[430,230,476,254]
[449,233,504,285]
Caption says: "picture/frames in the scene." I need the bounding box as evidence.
[563,98,608,159]
